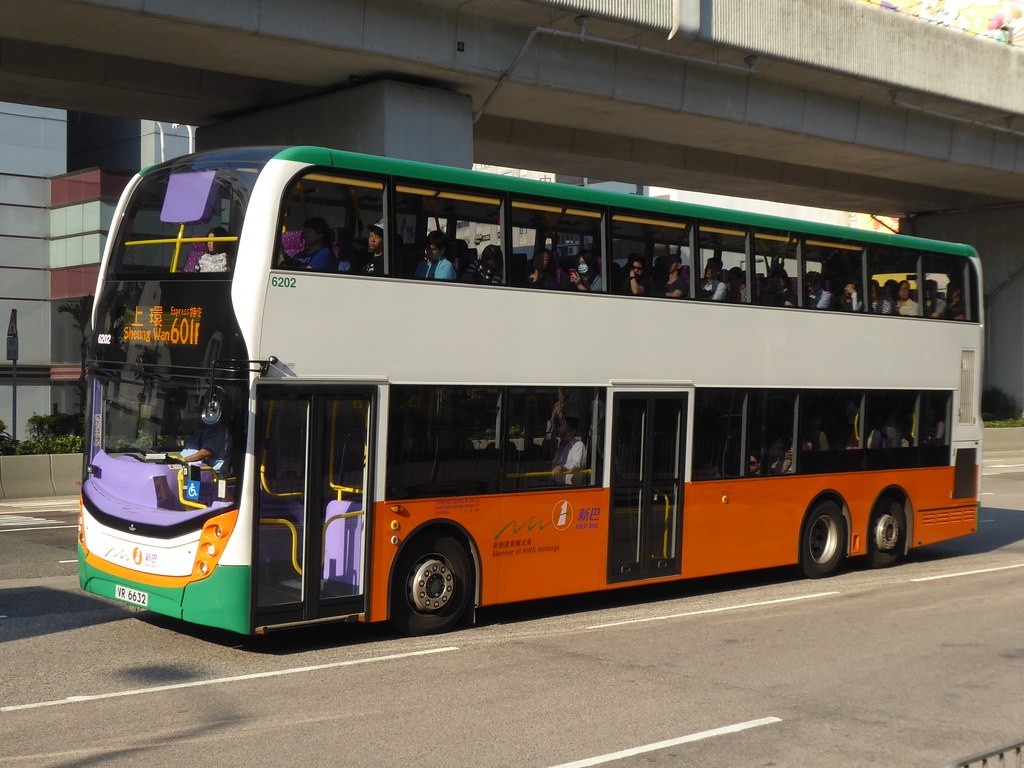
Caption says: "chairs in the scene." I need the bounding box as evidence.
[183,228,947,318]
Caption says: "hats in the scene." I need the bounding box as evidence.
[367,223,383,236]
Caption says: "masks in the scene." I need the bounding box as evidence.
[578,264,589,273]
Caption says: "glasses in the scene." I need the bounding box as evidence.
[746,461,757,466]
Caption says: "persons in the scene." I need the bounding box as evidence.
[391,409,942,479]
[181,390,237,480]
[192,227,232,273]
[277,215,970,323]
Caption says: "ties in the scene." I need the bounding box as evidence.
[198,426,204,449]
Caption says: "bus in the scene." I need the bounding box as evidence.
[74,142,985,641]
